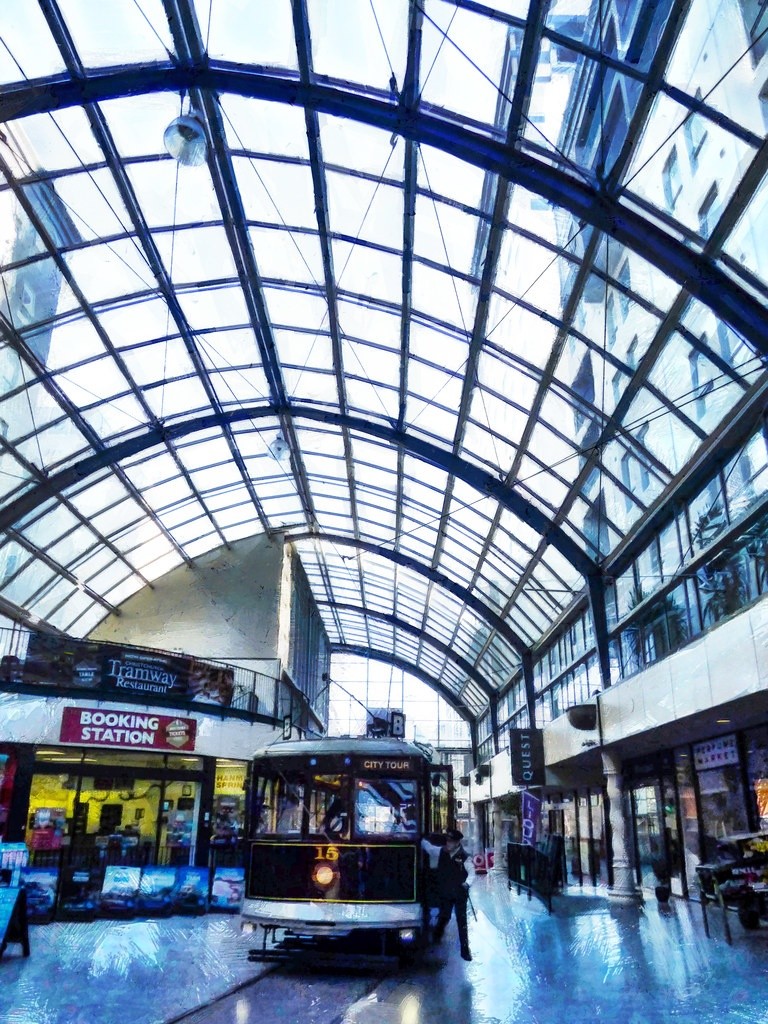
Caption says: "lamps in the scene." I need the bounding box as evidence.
[268,422,291,460]
[163,110,209,167]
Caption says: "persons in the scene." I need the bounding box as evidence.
[420,827,474,961]
[120,802,248,846]
[187,662,234,706]
[709,763,749,835]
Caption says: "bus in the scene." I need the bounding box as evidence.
[241,731,457,969]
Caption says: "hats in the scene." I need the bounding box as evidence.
[445,829,464,841]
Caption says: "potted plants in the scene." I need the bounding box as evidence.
[652,857,673,902]
[739,900,759,929]
